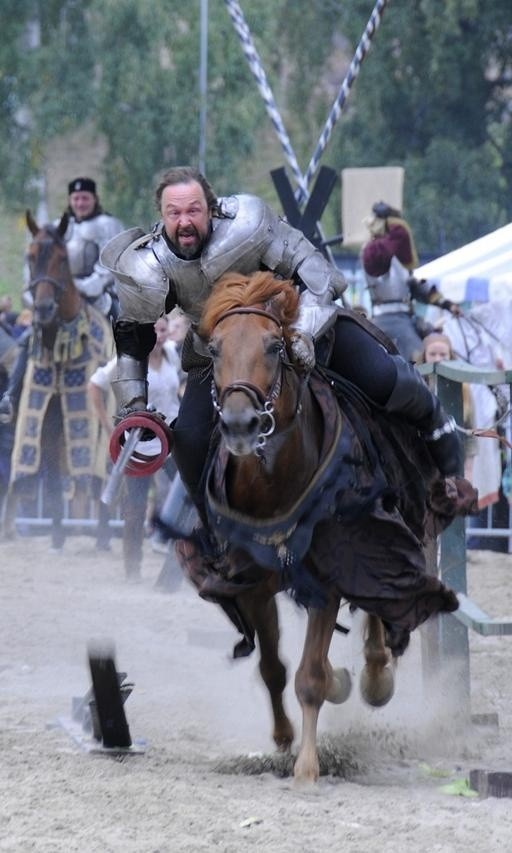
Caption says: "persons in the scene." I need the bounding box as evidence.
[0,176,131,423]
[415,330,474,432]
[0,292,190,594]
[103,165,461,476]
[361,200,461,362]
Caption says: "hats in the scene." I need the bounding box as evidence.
[68,177,96,193]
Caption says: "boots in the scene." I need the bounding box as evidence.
[381,356,466,474]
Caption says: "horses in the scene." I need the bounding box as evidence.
[1,209,118,556]
[196,271,424,798]
